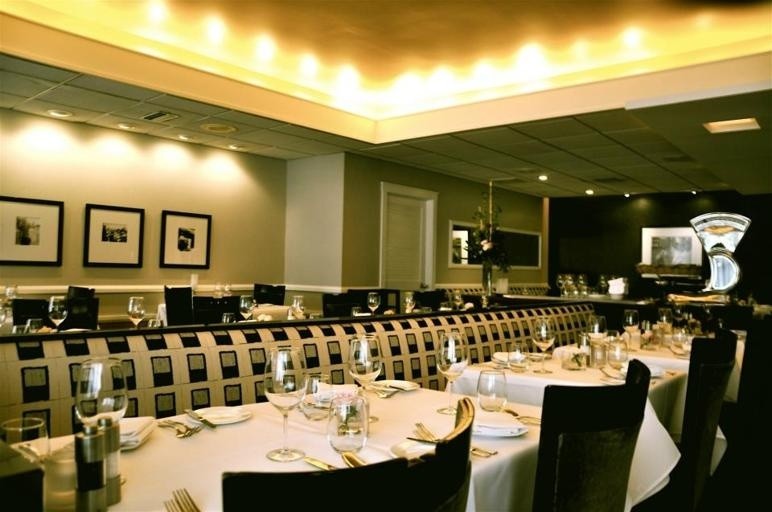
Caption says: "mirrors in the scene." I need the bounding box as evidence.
[445,218,543,273]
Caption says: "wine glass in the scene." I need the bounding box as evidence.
[346,333,384,426]
[76,357,129,485]
[185,272,327,328]
[555,272,620,299]
[345,286,491,318]
[263,346,308,462]
[0,281,68,334]
[437,332,468,415]
[493,302,717,375]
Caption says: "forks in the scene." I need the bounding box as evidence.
[504,409,541,422]
[158,419,207,440]
[162,488,201,511]
[405,421,500,458]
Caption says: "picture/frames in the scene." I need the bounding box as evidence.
[158,208,213,270]
[639,226,705,283]
[80,200,147,270]
[0,196,65,270]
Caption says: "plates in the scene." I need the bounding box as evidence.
[363,378,421,392]
[194,405,253,425]
[467,414,526,441]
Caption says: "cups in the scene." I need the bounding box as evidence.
[0,416,52,470]
[299,373,333,420]
[122,292,164,330]
[326,396,368,454]
[477,368,506,413]
[40,455,75,512]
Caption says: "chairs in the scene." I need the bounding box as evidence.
[2,270,771,512]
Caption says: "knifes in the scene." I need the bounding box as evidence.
[305,456,340,470]
[182,409,217,430]
